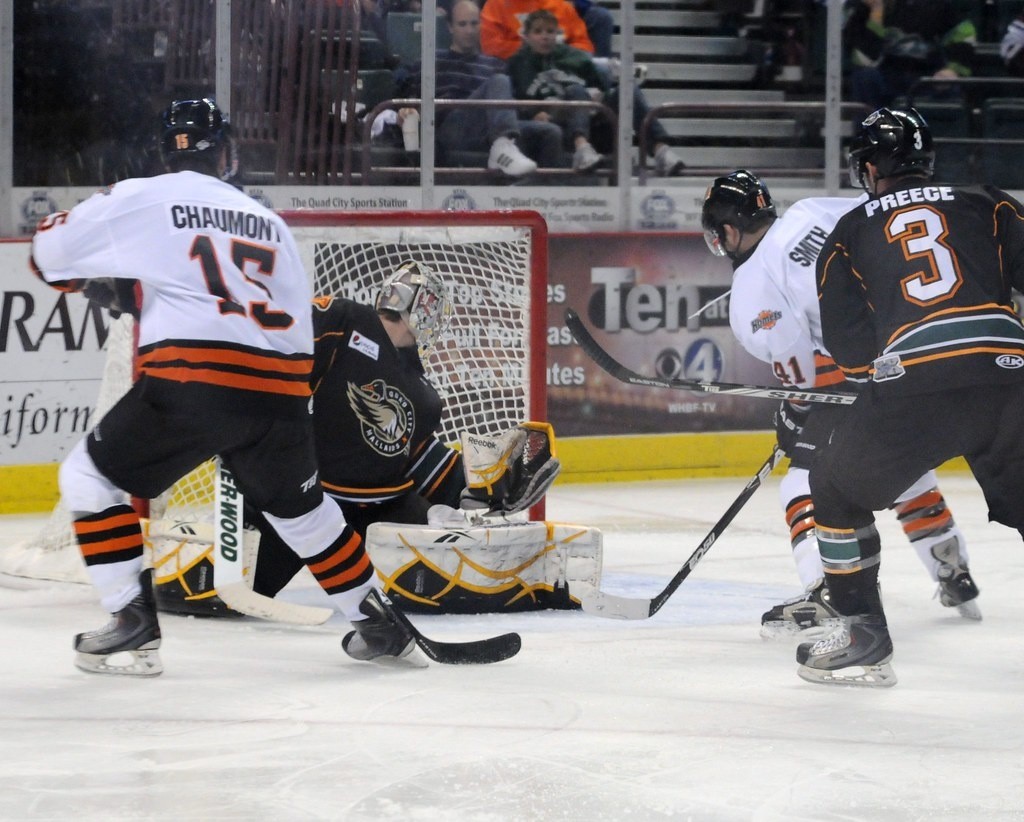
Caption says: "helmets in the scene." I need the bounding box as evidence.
[376,260,452,371]
[702,170,774,262]
[160,96,238,181]
[851,107,935,178]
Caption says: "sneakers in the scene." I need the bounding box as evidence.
[653,142,685,175]
[760,580,881,640]
[796,616,898,687]
[930,536,984,620]
[488,138,537,175]
[73,567,164,676]
[342,587,430,670]
[572,144,604,172]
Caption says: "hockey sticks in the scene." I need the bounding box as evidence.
[377,586,522,666]
[563,305,860,405]
[580,446,787,622]
[212,454,334,626]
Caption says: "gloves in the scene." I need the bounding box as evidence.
[79,275,128,315]
[776,399,811,452]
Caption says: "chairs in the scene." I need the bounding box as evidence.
[889,79,1024,193]
[266,0,640,157]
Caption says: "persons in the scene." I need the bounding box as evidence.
[155,258,560,618]
[843,0,1023,183]
[30,98,430,678]
[700,169,983,640]
[795,107,1023,690]
[389,0,687,176]
